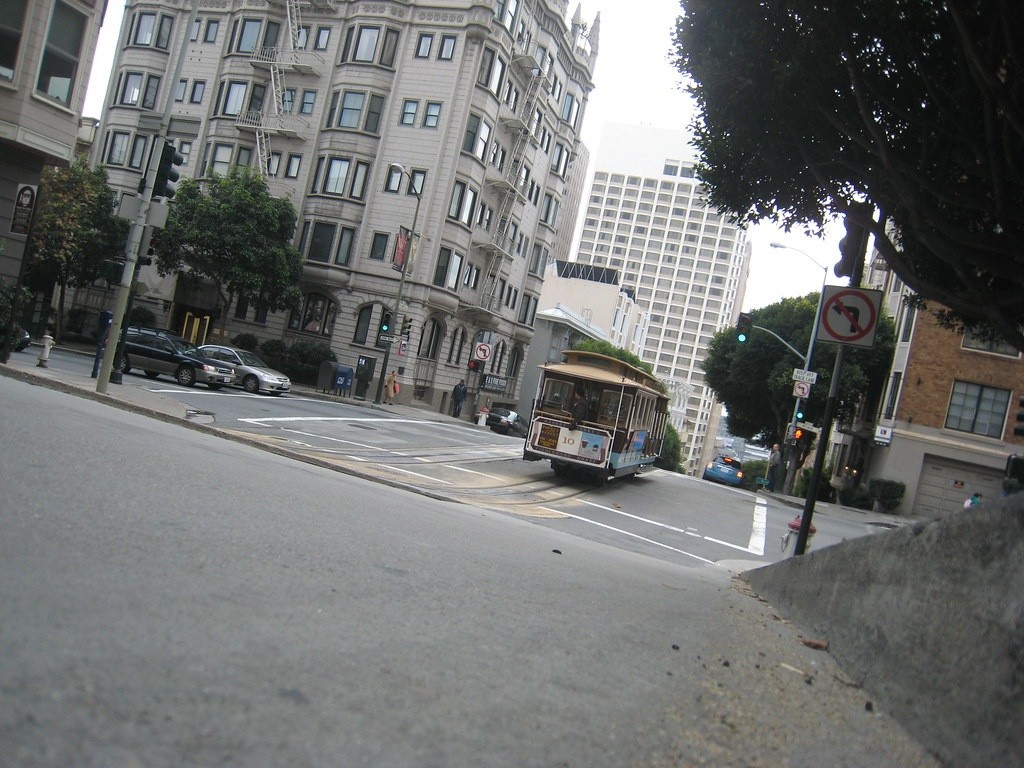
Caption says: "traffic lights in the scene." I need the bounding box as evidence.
[784,424,802,446]
[139,237,157,266]
[735,313,753,347]
[1004,454,1024,484]
[1014,395,1024,438]
[834,202,868,278]
[154,141,184,199]
[468,360,479,372]
[380,311,393,334]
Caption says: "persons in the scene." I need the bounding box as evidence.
[383,371,397,405]
[569,387,590,430]
[963,493,983,513]
[451,380,467,418]
[764,444,780,493]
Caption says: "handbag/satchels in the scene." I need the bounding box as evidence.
[964,498,972,509]
[394,383,399,394]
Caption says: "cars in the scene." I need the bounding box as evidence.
[474,407,528,438]
[701,456,745,488]
[13,324,31,353]
[182,345,292,396]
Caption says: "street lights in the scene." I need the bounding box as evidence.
[770,242,831,496]
[373,162,422,408]
[797,411,806,422]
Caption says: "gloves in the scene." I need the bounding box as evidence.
[569,423,576,431]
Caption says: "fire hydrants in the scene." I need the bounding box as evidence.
[35,330,56,369]
[780,515,817,560]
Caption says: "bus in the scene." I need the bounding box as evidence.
[521,350,671,489]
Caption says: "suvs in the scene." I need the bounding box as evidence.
[103,326,236,391]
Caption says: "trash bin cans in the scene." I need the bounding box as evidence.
[316,361,353,398]
